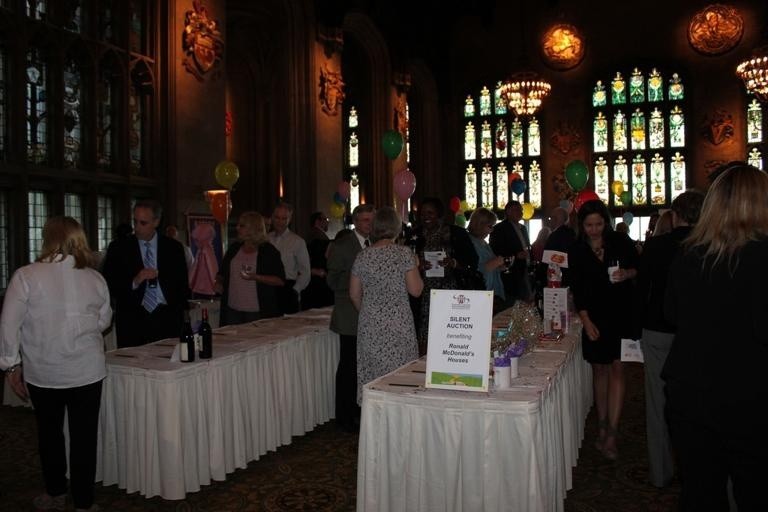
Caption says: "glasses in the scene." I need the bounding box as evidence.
[321,216,329,223]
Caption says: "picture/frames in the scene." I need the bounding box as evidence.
[182,213,224,301]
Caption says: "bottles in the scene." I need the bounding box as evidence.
[197,307,212,359]
[180,313,196,362]
[493,349,518,387]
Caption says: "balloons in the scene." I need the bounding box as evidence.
[219,159,634,226]
[380,127,404,161]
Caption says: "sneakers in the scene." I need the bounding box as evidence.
[75,504,100,512]
[32,494,68,512]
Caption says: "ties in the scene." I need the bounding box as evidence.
[364,240,368,247]
[143,241,158,314]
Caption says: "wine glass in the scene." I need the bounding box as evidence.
[491,299,549,355]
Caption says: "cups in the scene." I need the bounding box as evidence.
[147,266,158,287]
[240,261,253,280]
[607,260,621,282]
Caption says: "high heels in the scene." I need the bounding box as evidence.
[602,436,619,461]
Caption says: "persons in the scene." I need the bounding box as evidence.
[327,201,638,458]
[98,202,335,350]
[1,216,112,511]
[638,161,767,510]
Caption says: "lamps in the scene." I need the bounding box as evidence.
[734,43,768,107]
[499,62,551,129]
